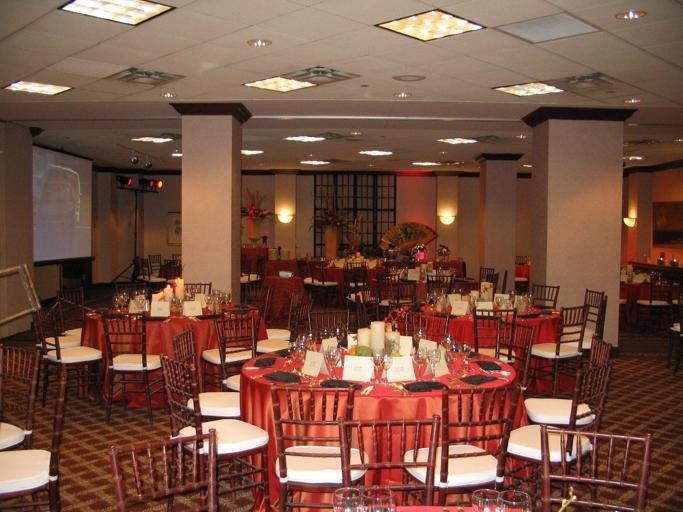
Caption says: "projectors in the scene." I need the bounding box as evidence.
[279,270,294,278]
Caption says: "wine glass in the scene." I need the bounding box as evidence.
[112,288,229,318]
[287,328,345,386]
[412,328,472,385]
[380,357,393,387]
[425,287,534,317]
[370,353,384,385]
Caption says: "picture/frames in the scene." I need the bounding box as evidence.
[166,211,181,246]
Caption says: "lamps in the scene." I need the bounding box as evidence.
[437,215,456,225]
[115,150,163,192]
[276,213,294,223]
[623,218,635,228]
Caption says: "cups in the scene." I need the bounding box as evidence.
[472,488,532,512]
[332,488,394,512]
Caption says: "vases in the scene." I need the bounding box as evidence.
[325,226,342,258]
[245,215,263,245]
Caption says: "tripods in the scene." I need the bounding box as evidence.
[113,196,141,284]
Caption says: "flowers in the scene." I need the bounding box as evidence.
[345,210,363,250]
[241,187,276,224]
[307,207,350,234]
